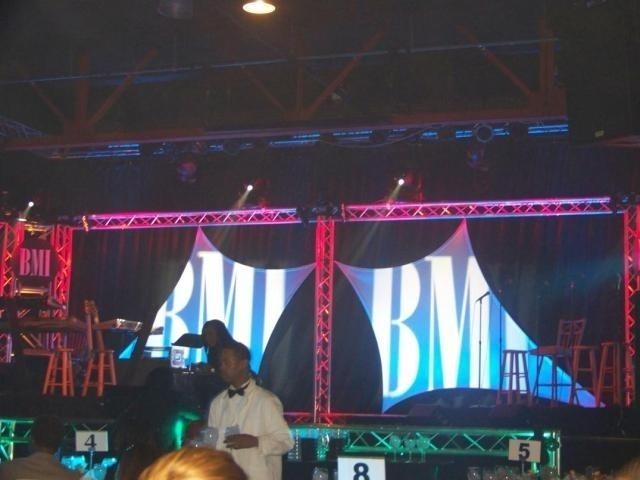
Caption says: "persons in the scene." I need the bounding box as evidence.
[0,412,81,480]
[210,340,294,480]
[192,319,258,408]
[114,396,160,480]
[136,445,248,480]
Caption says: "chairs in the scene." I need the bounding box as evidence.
[530,319,585,407]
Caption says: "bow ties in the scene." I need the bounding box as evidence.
[228,382,250,398]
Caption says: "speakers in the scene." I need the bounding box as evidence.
[406,404,640,438]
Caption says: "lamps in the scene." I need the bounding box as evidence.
[240,0,277,15]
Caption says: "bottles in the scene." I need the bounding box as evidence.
[287,428,303,460]
[467,459,617,480]
[87,448,95,471]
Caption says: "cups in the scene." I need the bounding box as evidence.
[194,426,221,451]
[315,432,330,458]
[314,467,329,479]
[225,424,238,450]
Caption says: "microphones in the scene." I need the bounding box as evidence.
[538,280,549,299]
[570,280,576,307]
[497,289,503,305]
[615,273,621,292]
[475,291,490,302]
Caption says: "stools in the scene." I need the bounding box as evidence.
[596,341,622,406]
[496,349,532,407]
[569,344,599,405]
[41,348,74,396]
[81,350,117,398]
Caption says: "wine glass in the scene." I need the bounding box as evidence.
[390,438,430,463]
